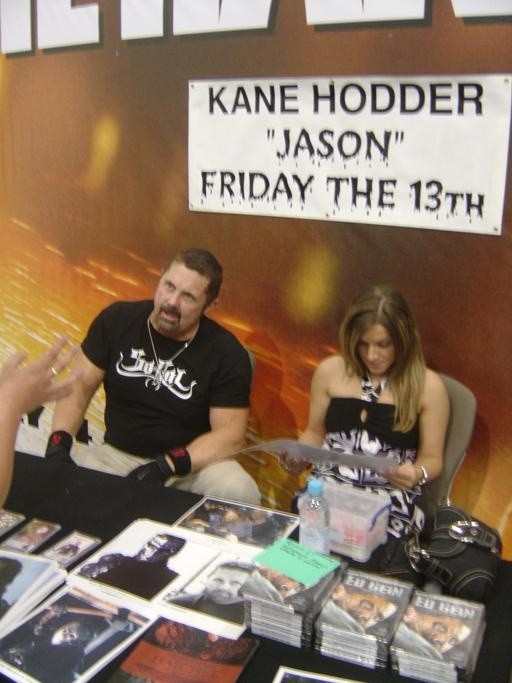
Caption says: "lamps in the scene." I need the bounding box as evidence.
[415,370,477,516]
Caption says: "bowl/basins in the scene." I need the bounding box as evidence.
[144,316,195,392]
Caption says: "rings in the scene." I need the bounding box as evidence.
[50,365,59,378]
[276,456,286,463]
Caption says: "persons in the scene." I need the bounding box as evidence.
[169,563,256,627]
[1,333,83,511]
[275,284,452,542]
[42,244,264,508]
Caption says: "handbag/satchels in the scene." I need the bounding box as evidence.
[167,445,192,479]
[46,429,72,453]
[419,464,430,488]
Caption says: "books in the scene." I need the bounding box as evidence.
[0,493,488,682]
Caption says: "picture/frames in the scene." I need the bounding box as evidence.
[0,450,510,681]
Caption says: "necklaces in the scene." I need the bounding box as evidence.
[144,316,195,392]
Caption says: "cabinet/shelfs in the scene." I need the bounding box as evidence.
[297,480,329,553]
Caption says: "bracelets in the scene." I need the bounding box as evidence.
[46,429,72,453]
[167,445,192,479]
[419,464,430,488]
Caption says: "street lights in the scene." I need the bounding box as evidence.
[399,497,502,601]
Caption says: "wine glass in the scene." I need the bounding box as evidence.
[50,365,59,378]
[276,456,286,463]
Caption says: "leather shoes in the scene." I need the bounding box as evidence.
[46,455,85,494]
[126,455,172,492]
